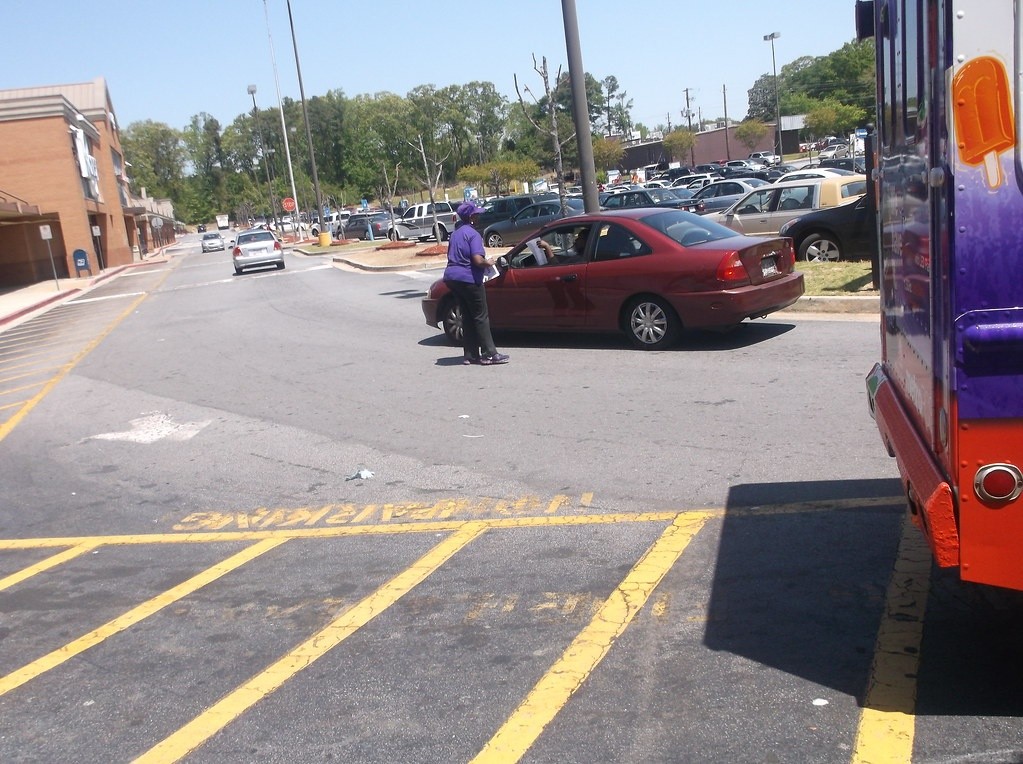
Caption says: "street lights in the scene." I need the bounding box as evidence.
[764,31,783,161]
[246,84,282,242]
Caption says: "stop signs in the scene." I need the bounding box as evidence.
[281,197,295,211]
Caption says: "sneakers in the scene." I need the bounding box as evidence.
[463,354,481,364]
[480,352,510,365]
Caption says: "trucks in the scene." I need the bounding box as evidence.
[214,214,230,230]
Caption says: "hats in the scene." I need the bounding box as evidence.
[457,201,485,219]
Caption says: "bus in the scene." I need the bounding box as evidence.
[835,1,1023,592]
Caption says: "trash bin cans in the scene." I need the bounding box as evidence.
[131,245,140,263]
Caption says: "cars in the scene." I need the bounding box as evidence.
[883,152,931,315]
[228,229,286,274]
[817,144,851,162]
[748,151,781,167]
[197,223,206,233]
[199,233,227,253]
[799,136,850,152]
[778,193,870,264]
[480,198,610,248]
[419,206,807,352]
[251,159,866,250]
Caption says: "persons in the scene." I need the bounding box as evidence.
[444,201,509,364]
[536,229,589,265]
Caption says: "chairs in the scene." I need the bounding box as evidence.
[782,198,801,210]
[540,208,550,215]
[636,196,647,204]
[598,226,636,259]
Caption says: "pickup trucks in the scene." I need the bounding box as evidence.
[668,176,867,243]
